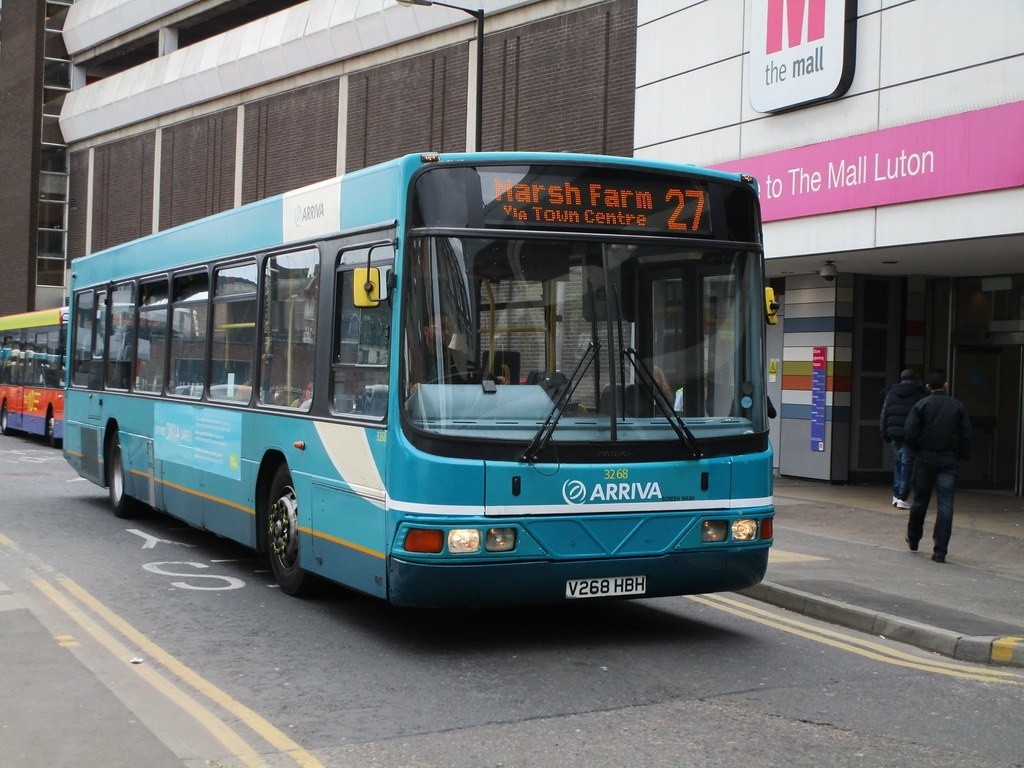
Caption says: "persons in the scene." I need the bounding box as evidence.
[901,368,973,564]
[407,311,510,396]
[879,369,927,509]
[653,365,673,417]
[673,385,683,417]
[289,380,314,408]
[729,396,776,418]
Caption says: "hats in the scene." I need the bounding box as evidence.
[901,369,918,378]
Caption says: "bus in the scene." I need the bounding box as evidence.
[59,148,783,614]
[0,303,200,448]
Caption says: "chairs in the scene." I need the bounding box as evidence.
[598,382,634,414]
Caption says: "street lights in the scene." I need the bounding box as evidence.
[397,0,486,152]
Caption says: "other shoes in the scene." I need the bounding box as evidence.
[905,535,918,551]
[892,496,898,505]
[932,554,945,562]
[897,500,911,508]
